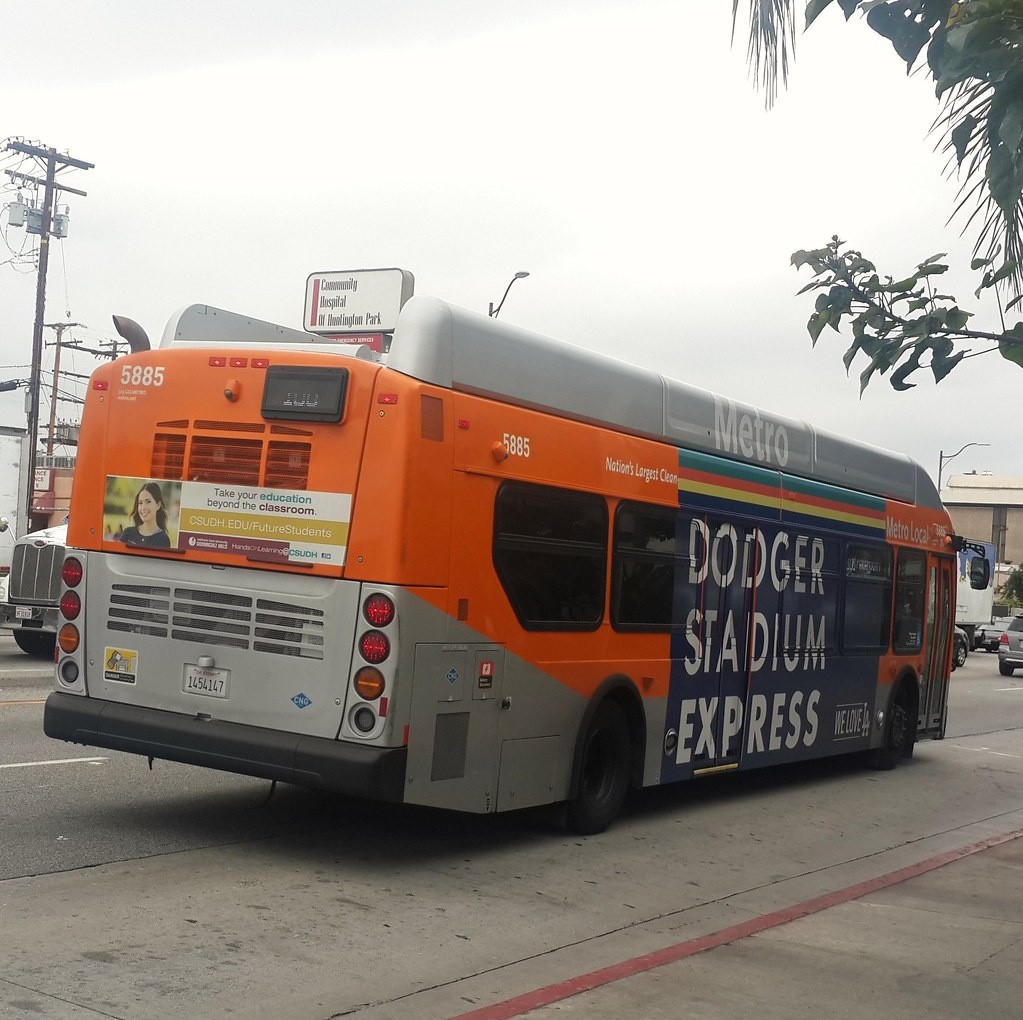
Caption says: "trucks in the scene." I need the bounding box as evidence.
[0,516,67,657]
[956,538,997,650]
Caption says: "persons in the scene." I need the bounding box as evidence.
[104,524,122,540]
[119,482,170,548]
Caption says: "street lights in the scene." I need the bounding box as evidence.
[997,527,1009,597]
[489,270,528,316]
[938,443,991,495]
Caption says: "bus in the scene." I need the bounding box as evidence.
[43,267,964,832]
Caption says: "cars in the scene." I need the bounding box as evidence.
[974,615,1015,652]
[951,626,970,671]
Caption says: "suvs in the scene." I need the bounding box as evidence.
[998,613,1023,675]
